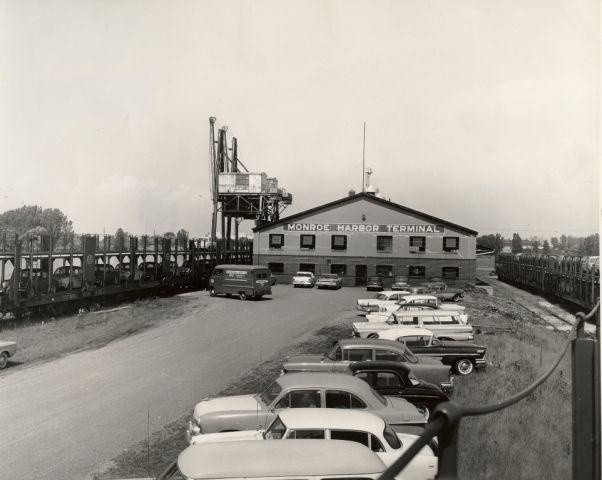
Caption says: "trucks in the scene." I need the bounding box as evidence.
[208,264,272,300]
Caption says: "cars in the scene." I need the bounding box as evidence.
[176,337,455,480]
[292,271,315,288]
[351,275,488,375]
[316,274,344,290]
[0,340,18,368]
[515,252,600,276]
[0,258,253,298]
